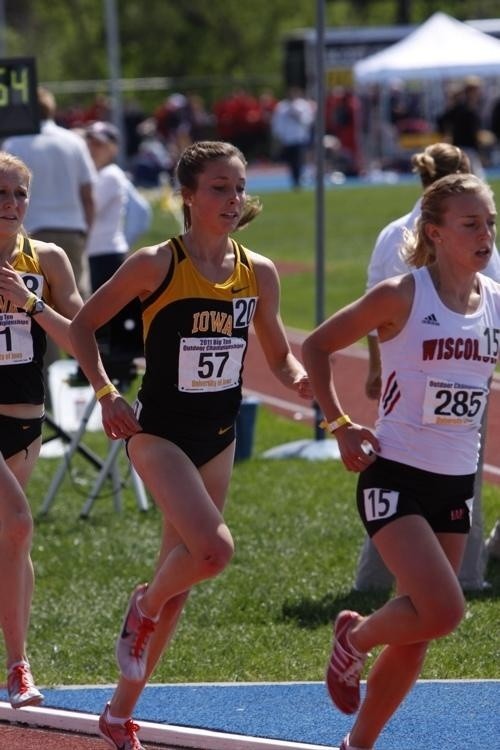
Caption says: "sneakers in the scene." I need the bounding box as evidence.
[326,611,367,714]
[97,703,145,749]
[116,584,159,682]
[8,658,43,709]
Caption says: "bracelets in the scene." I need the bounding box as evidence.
[23,293,37,311]
[317,410,350,434]
[94,384,118,400]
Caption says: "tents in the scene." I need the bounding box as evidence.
[353,12,499,108]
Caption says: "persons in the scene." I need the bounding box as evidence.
[298,174,500,750]
[0,153,85,708]
[356,142,498,600]
[65,141,317,750]
[65,119,138,396]
[53,76,499,190]
[2,86,96,413]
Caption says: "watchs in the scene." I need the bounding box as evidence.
[25,301,45,317]
